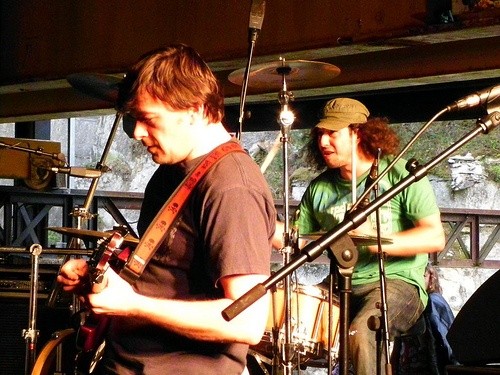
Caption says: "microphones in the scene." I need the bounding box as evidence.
[248,0,267,43]
[447,85,500,111]
[44,166,102,178]
[355,149,381,211]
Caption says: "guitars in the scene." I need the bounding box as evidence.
[73,230,125,375]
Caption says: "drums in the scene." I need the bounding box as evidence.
[247,279,343,366]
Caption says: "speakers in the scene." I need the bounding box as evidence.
[445,270,500,365]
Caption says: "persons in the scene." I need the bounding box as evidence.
[271,98,446,375]
[54,43,278,375]
[388,262,459,375]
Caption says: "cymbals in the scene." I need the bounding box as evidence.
[227,57,342,90]
[298,230,394,248]
[44,224,140,246]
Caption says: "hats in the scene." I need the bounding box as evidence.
[314,98,370,131]
